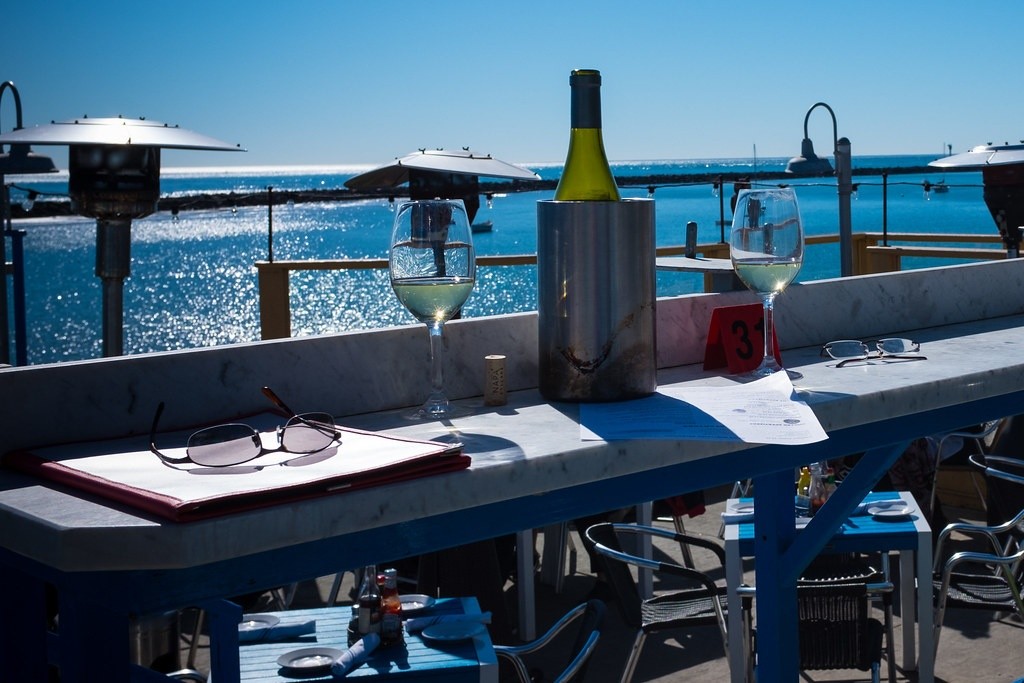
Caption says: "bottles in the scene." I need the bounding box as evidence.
[797,462,837,516]
[346,563,401,652]
[555,68,620,200]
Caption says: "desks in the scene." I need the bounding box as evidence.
[656,254,749,292]
[207,594,499,683]
[724,489,933,683]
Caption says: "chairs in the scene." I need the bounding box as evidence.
[494,406,1024,683]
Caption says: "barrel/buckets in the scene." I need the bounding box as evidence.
[535,198,658,404]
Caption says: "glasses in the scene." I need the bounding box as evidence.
[820,337,927,368]
[150,387,341,467]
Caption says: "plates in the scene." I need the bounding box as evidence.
[277,647,344,668]
[398,593,435,613]
[729,502,754,513]
[421,620,486,642]
[239,614,279,630]
[867,505,916,517]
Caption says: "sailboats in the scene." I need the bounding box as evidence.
[931,140,951,193]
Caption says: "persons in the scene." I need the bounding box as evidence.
[376,489,705,607]
[821,423,986,547]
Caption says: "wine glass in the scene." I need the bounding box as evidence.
[387,199,475,421]
[729,188,804,382]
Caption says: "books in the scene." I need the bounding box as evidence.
[42,418,470,524]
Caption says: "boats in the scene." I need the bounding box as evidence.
[470,219,493,234]
